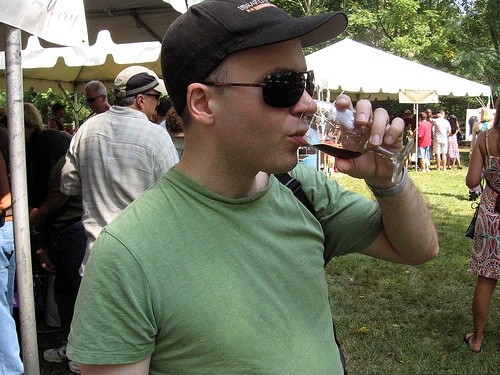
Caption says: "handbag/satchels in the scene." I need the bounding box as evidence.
[465,202,479,240]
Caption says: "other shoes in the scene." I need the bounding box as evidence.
[37,322,63,333]
[420,168,462,172]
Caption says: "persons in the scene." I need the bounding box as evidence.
[402,108,463,172]
[67,0,439,375]
[464,96,500,352]
[0,65,185,375]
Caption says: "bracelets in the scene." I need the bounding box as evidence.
[368,166,408,197]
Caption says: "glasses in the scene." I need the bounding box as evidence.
[198,70,315,108]
[135,94,160,100]
[85,95,103,103]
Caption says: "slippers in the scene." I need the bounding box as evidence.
[465,332,482,354]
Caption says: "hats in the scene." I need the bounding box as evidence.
[52,103,66,112]
[113,66,167,97]
[161,0,348,117]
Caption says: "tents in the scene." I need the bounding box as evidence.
[0,0,196,375]
[304,38,493,172]
[0,30,167,133]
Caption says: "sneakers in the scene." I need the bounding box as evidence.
[44,345,67,362]
[68,360,80,373]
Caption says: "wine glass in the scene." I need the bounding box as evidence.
[296,99,415,183]
[29,207,39,235]
